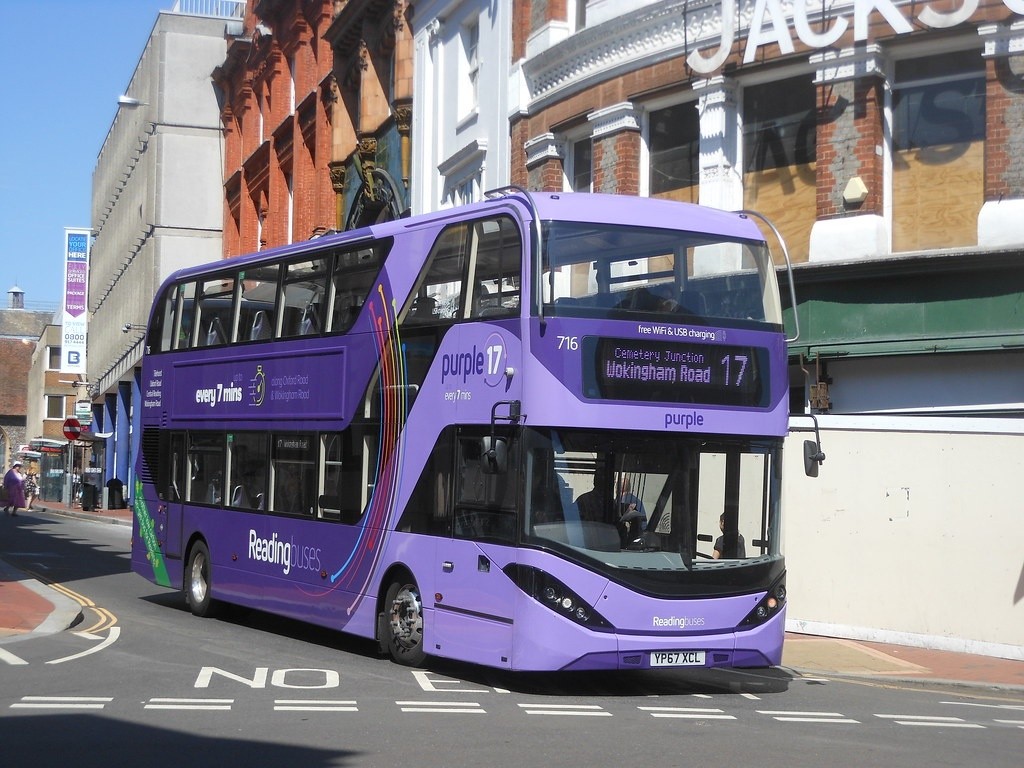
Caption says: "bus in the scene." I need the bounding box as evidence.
[132,184,825,673]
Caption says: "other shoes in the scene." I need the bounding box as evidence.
[4,508,9,513]
[12,513,18,516]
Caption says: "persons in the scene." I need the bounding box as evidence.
[614,476,647,531]
[72,465,80,502]
[562,468,626,550]
[4,461,41,516]
[713,512,745,559]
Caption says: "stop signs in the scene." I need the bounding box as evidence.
[62,418,81,440]
